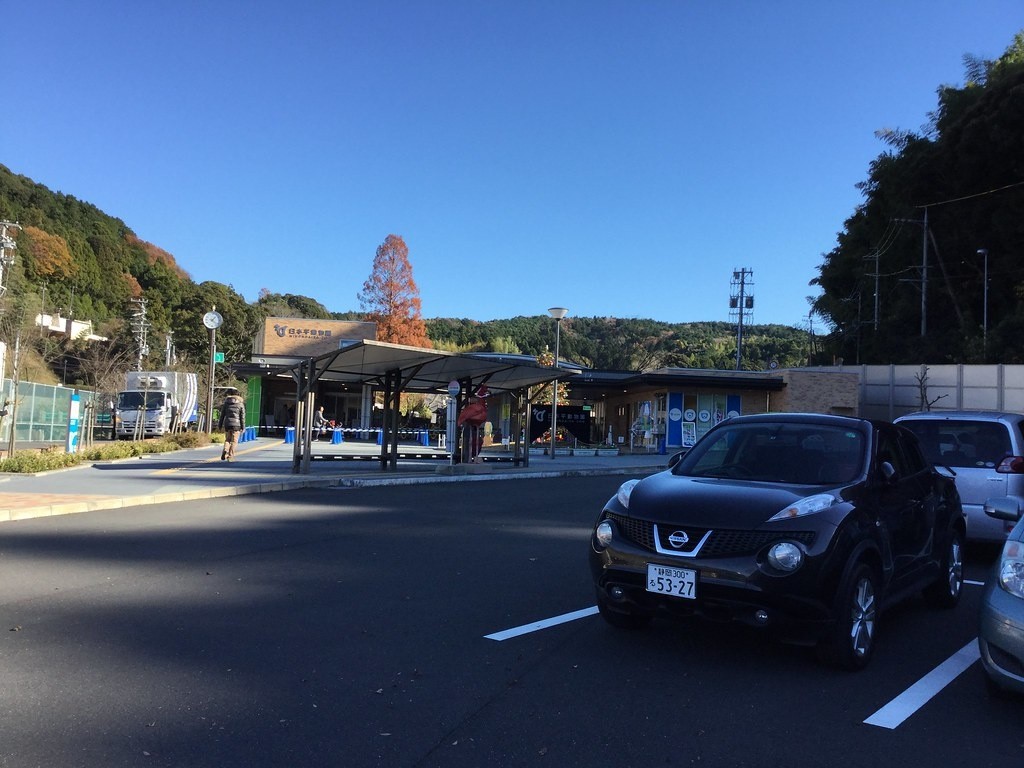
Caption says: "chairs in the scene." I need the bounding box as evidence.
[942,451,963,466]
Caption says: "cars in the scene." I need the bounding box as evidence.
[589,412,967,669]
[977,493,1023,698]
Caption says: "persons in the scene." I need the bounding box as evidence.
[218,389,245,460]
[313,406,328,442]
[283,400,295,420]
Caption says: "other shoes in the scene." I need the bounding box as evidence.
[313,439,319,442]
[221,451,227,460]
[228,457,233,462]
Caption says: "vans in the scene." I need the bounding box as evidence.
[891,410,1024,543]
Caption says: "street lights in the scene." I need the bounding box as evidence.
[547,307,569,460]
[977,248,988,363]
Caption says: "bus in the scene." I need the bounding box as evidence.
[211,387,242,402]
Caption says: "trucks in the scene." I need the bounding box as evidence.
[115,372,199,441]
